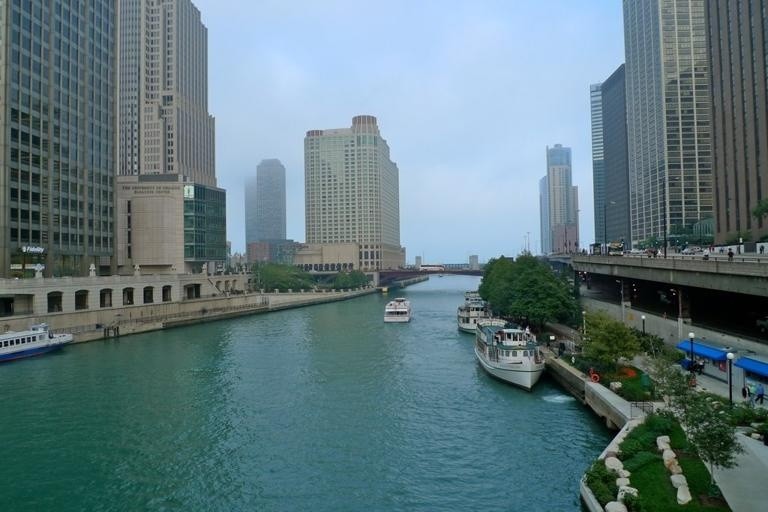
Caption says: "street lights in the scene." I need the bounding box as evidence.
[604,200,616,254]
[641,315,646,334]
[575,209,582,253]
[615,279,626,323]
[662,176,680,258]
[522,219,572,254]
[727,352,734,405]
[582,310,586,338]
[669,288,684,344]
[688,331,694,372]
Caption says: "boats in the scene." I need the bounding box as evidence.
[456,289,494,335]
[475,315,547,394]
[383,297,413,323]
[0,322,75,362]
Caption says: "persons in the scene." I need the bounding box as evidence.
[102,325,120,337]
[727,247,734,261]
[755,380,765,405]
[741,382,752,404]
[662,311,668,323]
[747,382,756,405]
[702,249,709,261]
[759,244,766,256]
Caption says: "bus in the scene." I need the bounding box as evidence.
[590,241,624,256]
[419,264,448,272]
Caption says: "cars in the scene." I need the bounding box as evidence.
[627,248,657,255]
[681,246,702,256]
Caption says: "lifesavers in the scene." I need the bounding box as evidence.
[591,374,599,383]
